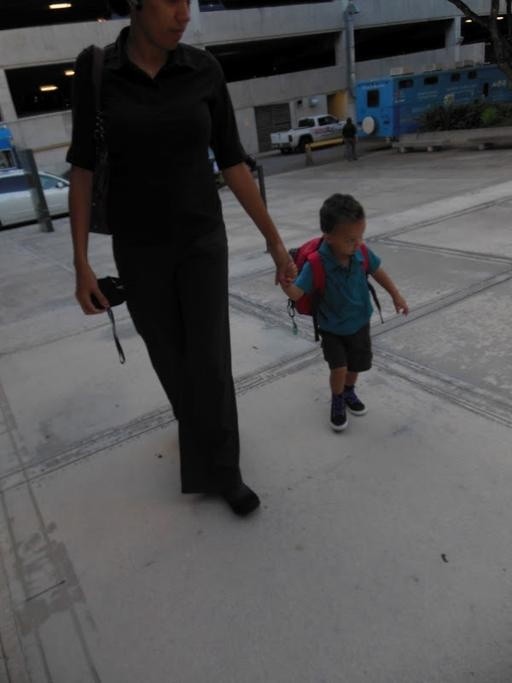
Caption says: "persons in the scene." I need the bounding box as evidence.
[273,189,414,431]
[63,0,299,519]
[340,115,360,165]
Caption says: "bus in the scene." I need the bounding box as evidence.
[354,61,512,140]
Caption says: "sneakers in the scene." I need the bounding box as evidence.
[220,481,260,516]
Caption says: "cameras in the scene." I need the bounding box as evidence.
[91,275,125,310]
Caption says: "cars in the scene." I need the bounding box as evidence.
[1,167,71,231]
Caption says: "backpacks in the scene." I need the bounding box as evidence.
[288,237,369,316]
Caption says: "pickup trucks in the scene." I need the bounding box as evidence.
[269,112,346,153]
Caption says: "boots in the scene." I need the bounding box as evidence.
[344,385,368,416]
[330,391,348,432]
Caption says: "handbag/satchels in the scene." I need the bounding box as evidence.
[88,150,113,236]
